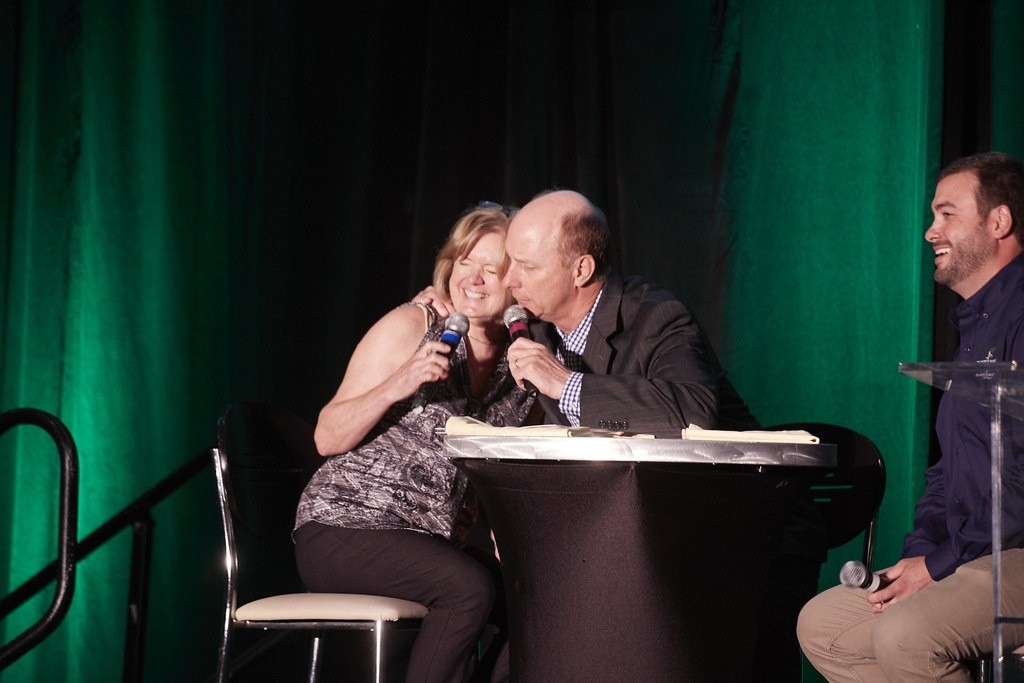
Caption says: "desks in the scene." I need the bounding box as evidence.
[444,434,822,682]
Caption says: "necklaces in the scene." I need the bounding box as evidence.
[467,334,498,346]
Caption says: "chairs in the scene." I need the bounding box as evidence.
[212,445,429,683]
[759,424,886,573]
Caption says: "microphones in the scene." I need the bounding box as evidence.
[839,561,895,601]
[411,312,470,414]
[502,304,541,397]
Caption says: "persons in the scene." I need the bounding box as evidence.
[797,149,1024,683]
[292,203,536,683]
[410,189,763,437]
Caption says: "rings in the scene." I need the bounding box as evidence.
[514,359,519,368]
